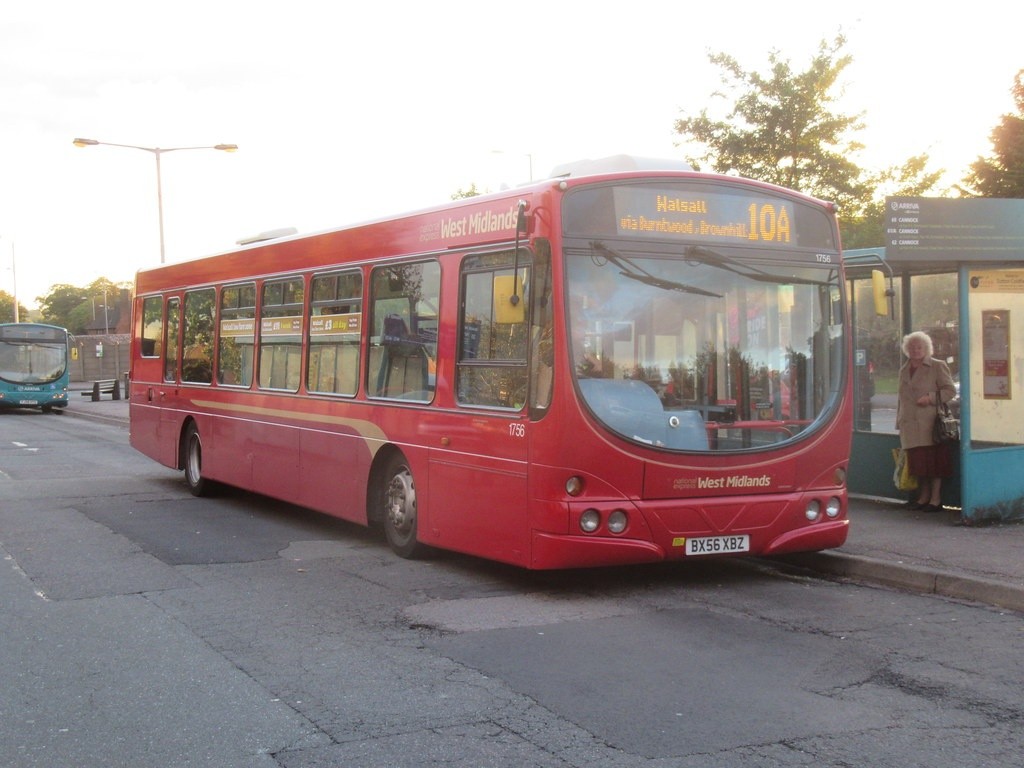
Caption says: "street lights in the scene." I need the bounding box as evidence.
[72,138,239,268]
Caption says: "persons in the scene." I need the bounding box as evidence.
[894,329,956,514]
[534,288,603,410]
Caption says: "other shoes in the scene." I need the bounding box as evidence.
[906,500,930,511]
[923,503,941,513]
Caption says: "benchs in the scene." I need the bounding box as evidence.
[81,379,121,401]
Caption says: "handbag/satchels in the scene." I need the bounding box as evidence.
[933,390,960,443]
[892,447,919,491]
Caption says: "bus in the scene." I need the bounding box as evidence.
[127,169,895,572]
[1,322,78,413]
